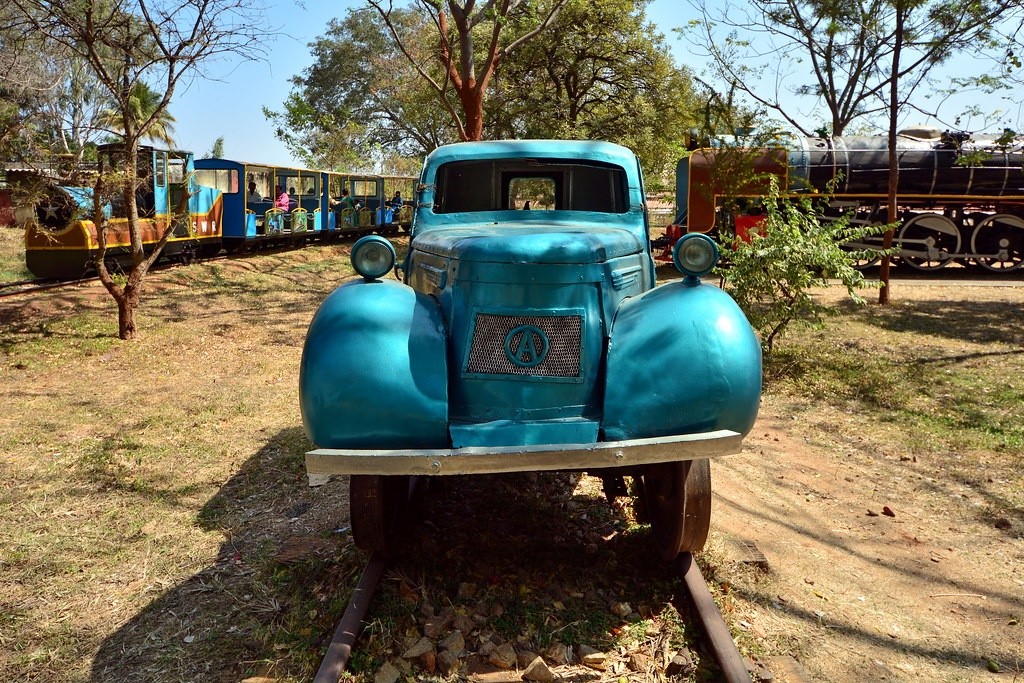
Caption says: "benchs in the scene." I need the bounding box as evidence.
[246,199,415,233]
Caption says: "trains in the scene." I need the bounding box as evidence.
[19,141,424,282]
[296,137,766,564]
[652,125,1024,277]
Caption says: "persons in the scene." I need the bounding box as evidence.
[321,188,332,198]
[331,188,349,230]
[307,188,314,196]
[289,187,296,195]
[389,191,403,222]
[247,182,261,202]
[353,197,361,211]
[272,185,290,213]
